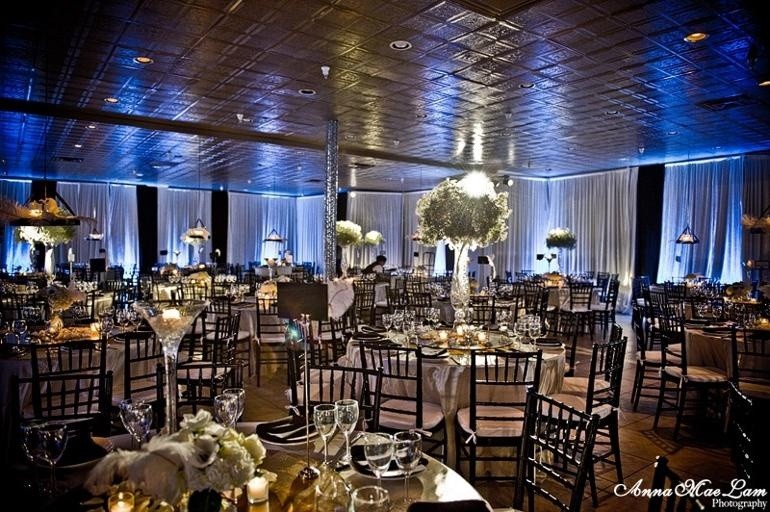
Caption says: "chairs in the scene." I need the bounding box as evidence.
[1,252,770,512]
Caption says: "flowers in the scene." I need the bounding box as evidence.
[364,230,383,247]
[545,227,577,250]
[13,226,77,248]
[336,220,362,249]
[411,178,513,294]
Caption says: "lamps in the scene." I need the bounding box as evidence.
[263,170,283,241]
[8,73,81,250]
[492,175,513,188]
[180,134,212,247]
[675,151,699,244]
[83,172,104,241]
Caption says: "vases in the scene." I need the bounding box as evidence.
[341,247,349,274]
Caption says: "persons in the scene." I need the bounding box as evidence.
[363,255,387,275]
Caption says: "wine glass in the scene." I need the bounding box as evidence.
[19,387,427,511]
[4,307,150,357]
[373,307,544,359]
